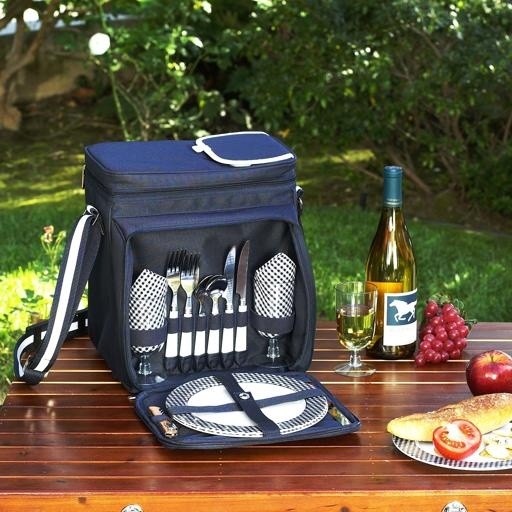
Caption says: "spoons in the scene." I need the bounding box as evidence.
[194,274,227,317]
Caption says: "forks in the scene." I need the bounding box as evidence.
[165,247,200,313]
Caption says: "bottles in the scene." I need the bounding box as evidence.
[365,165,419,360]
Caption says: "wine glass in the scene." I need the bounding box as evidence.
[253,281,296,369]
[128,296,167,386]
[333,281,378,378]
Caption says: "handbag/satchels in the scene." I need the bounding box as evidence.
[13,131,362,452]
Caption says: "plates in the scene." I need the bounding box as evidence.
[166,372,329,438]
[392,436,512,472]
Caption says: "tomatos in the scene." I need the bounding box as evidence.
[432,419,482,460]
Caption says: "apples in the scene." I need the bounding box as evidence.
[466,348,512,395]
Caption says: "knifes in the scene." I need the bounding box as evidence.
[305,381,352,426]
[221,240,251,311]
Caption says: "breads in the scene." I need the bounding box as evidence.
[386,392,512,441]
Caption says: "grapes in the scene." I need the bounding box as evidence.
[412,293,472,366]
